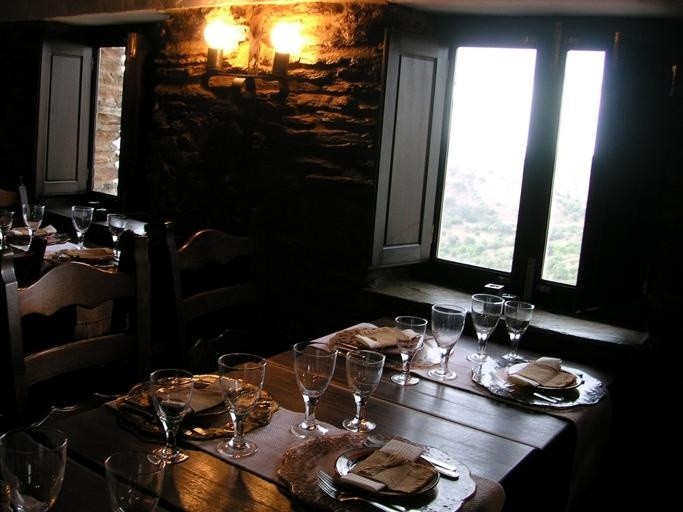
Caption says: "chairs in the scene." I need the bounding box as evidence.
[1,235,156,428]
[165,219,270,367]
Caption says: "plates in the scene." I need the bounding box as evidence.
[335,446,440,499]
[356,337,425,354]
[126,378,238,417]
[508,361,582,390]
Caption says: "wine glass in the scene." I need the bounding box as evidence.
[290,341,338,440]
[426,303,467,381]
[22,204,45,251]
[342,349,386,432]
[71,206,94,248]
[0,210,15,250]
[390,316,428,386]
[467,294,504,365]
[501,301,535,362]
[216,353,267,459]
[145,369,193,465]
[107,214,128,261]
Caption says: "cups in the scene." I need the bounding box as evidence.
[0,426,67,512]
[105,450,166,512]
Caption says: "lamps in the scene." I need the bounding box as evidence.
[199,19,302,101]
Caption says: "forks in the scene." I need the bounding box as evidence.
[364,435,459,481]
[488,366,565,404]
[316,470,407,512]
